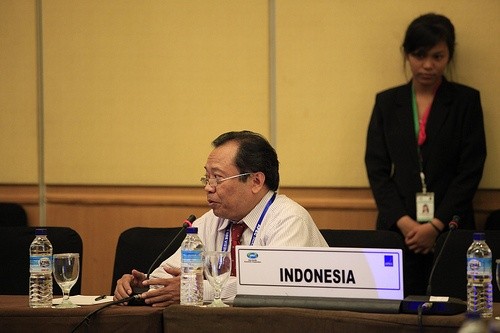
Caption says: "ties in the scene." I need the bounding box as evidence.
[230,224,248,276]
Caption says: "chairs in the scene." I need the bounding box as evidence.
[430,228,500,300]
[0,201,402,295]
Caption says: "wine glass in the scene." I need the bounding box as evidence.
[52,254,80,309]
[202,252,231,308]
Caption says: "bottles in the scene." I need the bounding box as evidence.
[29,229,52,308]
[179,228,206,307]
[466,232,493,321]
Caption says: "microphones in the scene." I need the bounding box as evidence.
[400,215,467,315]
[128,215,196,306]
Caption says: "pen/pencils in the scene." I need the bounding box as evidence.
[94,294,106,301]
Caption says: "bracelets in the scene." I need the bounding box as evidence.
[429,221,441,234]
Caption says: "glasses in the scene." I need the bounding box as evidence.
[200,172,251,186]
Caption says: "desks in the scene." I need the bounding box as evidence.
[0,296,500,333]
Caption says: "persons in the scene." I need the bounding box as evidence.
[114,130,329,309]
[366,12,487,302]
